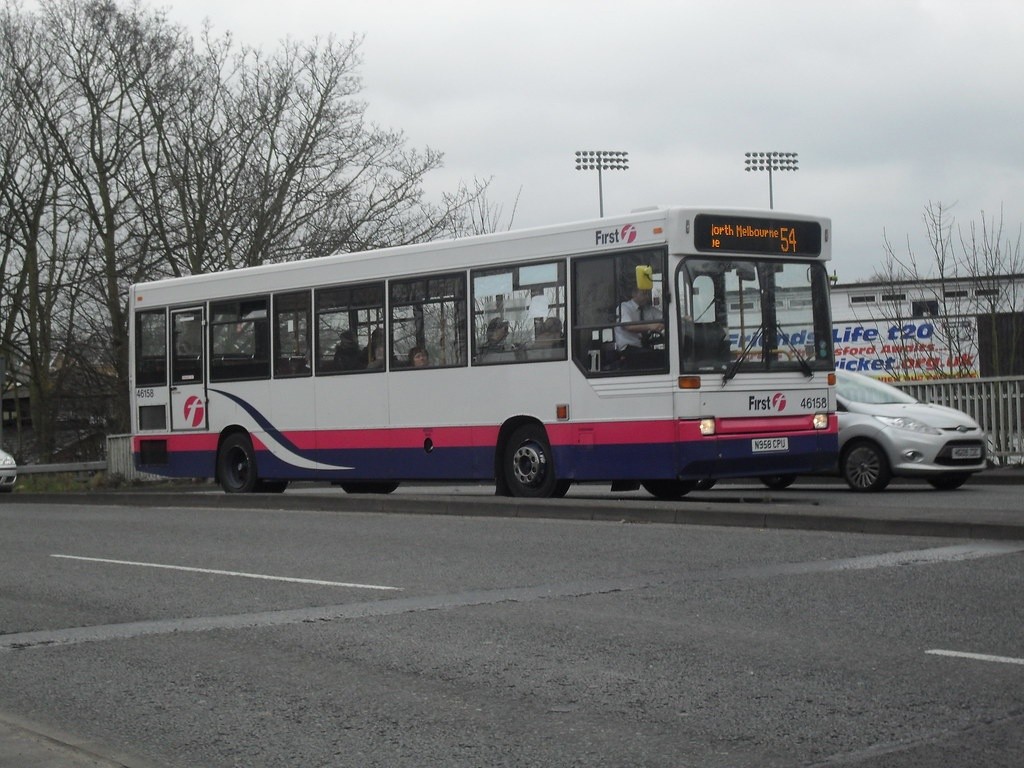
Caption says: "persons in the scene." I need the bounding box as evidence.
[479,316,513,353]
[530,317,567,350]
[612,279,664,351]
[408,346,430,367]
[332,329,386,372]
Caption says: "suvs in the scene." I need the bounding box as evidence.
[697,366,988,494]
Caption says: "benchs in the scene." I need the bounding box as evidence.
[141,352,337,379]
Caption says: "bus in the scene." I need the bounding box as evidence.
[129,206,837,499]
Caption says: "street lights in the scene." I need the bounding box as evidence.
[742,151,800,210]
[575,149,631,218]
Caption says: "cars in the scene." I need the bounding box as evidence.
[0,448,18,494]
